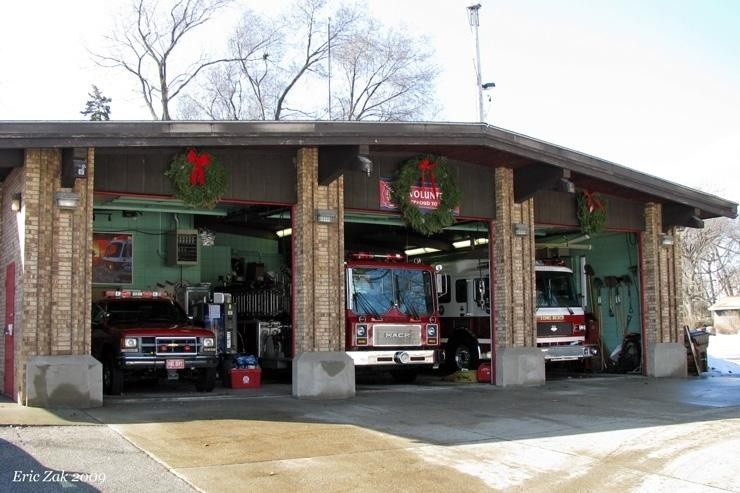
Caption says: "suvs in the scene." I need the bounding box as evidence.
[92,289,220,395]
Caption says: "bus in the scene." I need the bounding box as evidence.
[102,239,132,271]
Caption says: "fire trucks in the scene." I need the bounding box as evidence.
[430,256,602,377]
[208,250,448,381]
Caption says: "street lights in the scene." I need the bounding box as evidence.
[466,3,494,122]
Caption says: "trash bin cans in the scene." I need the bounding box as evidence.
[684,331,709,376]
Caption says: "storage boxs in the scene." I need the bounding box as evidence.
[229,365,262,389]
